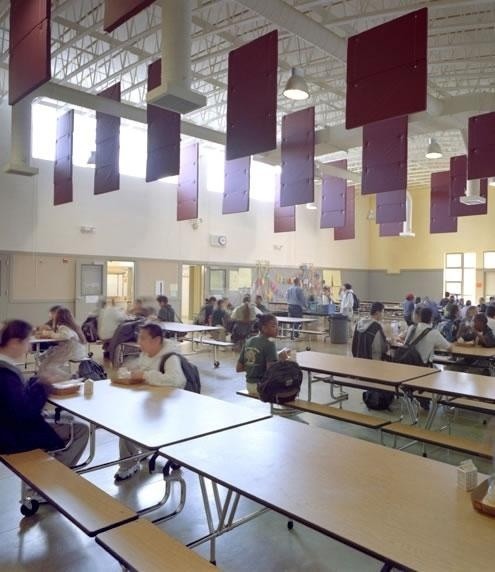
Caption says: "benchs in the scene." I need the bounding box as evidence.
[431,329,495,371]
[37,350,90,370]
[236,386,389,430]
[382,421,495,463]
[171,336,235,368]
[12,357,34,370]
[95,338,140,365]
[1,449,138,536]
[93,517,223,570]
[211,326,290,350]
[269,301,407,351]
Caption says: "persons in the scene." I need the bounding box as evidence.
[114,323,187,482]
[35,308,88,367]
[405,308,453,411]
[235,313,290,396]
[394,291,495,375]
[90,277,360,363]
[0,318,90,505]
[351,302,393,361]
[32,305,62,336]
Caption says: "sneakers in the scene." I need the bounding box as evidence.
[114,463,140,480]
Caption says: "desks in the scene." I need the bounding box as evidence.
[143,320,224,334]
[292,349,441,444]
[402,371,495,457]
[28,335,70,352]
[42,376,274,451]
[158,417,495,570]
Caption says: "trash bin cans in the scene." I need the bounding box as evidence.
[329,312,351,344]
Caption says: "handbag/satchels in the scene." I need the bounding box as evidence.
[160,352,201,392]
[363,389,393,411]
[78,359,106,380]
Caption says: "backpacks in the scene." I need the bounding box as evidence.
[393,324,431,366]
[346,291,358,309]
[258,361,303,403]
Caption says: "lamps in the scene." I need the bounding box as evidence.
[304,196,319,211]
[281,65,311,102]
[425,133,445,161]
[86,151,99,166]
